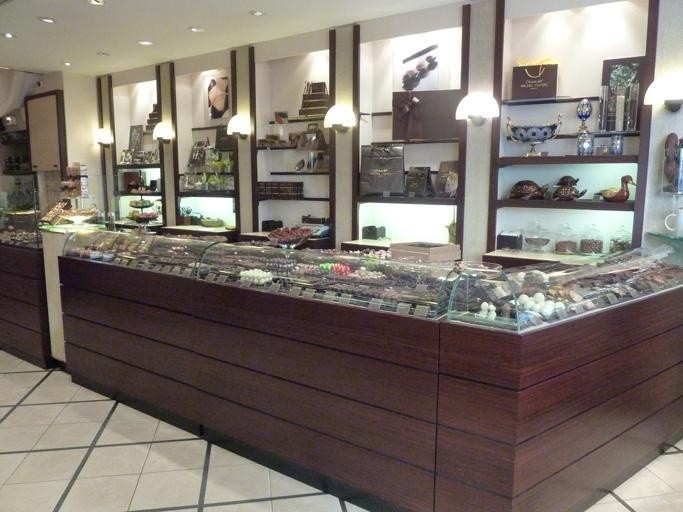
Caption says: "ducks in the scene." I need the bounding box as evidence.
[592,175,638,202]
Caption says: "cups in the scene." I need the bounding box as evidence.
[610,135,624,155]
[577,135,595,157]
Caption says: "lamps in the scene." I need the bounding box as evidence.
[455,92,500,127]
[153,120,176,144]
[94,127,114,148]
[324,103,357,133]
[643,72,683,112]
[227,114,252,139]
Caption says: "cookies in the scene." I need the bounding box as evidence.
[1,187,682,326]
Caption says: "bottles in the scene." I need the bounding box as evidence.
[580,223,604,256]
[609,225,632,256]
[554,223,577,254]
[525,220,551,253]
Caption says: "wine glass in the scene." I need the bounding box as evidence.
[267,229,314,280]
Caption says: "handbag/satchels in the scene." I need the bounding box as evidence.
[359,144,405,196]
[297,123,327,151]
[511,64,557,100]
